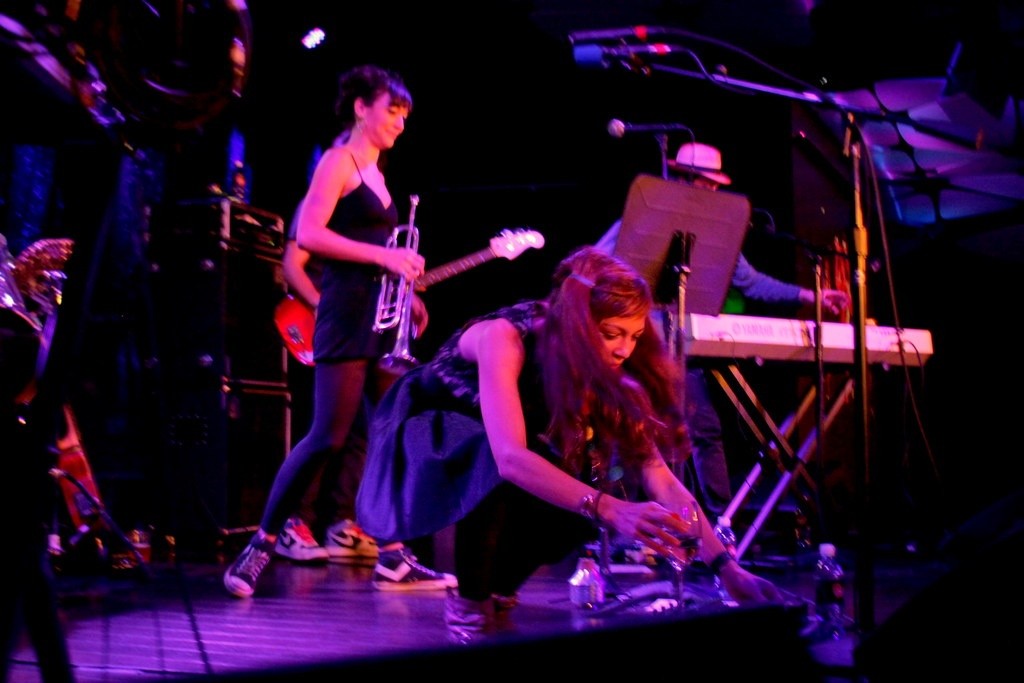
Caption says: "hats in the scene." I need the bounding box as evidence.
[665,141,733,186]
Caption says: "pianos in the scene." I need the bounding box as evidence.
[677,309,934,365]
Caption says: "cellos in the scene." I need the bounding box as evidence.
[15,380,111,550]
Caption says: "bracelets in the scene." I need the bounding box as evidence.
[710,551,735,576]
[579,491,605,520]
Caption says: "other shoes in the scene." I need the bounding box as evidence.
[443,586,518,625]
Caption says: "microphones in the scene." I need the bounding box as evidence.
[608,118,689,138]
[573,42,679,64]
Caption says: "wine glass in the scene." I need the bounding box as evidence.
[654,495,702,605]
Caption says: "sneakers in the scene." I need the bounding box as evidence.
[275,516,329,561]
[372,547,458,591]
[224,532,275,599]
[325,519,380,557]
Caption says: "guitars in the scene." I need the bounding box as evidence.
[273,228,545,367]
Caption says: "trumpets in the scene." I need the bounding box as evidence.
[372,192,423,375]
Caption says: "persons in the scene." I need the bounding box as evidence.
[595,143,847,565]
[222,66,458,599]
[357,246,783,633]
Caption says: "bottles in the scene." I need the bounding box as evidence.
[815,546,845,637]
[712,515,738,602]
